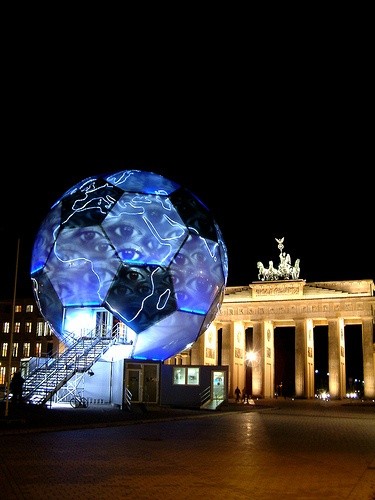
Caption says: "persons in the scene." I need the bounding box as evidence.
[245,387,251,403]
[9,371,26,402]
[242,387,247,402]
[235,387,241,403]
